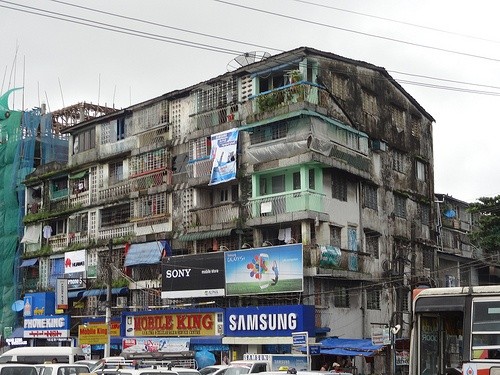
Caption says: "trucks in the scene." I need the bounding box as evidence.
[226,354,312,375]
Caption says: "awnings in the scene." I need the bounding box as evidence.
[83,287,129,296]
[178,229,233,241]
[68,290,85,297]
[20,258,37,266]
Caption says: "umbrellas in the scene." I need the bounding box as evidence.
[195,349,216,369]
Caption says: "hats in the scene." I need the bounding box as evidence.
[333,363,340,367]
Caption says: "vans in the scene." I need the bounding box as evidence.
[73,347,353,375]
[0,361,92,375]
[0,344,86,365]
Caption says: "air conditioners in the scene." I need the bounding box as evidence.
[116,297,127,308]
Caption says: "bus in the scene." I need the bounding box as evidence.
[389,285,500,375]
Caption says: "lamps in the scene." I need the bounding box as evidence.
[241,243,252,248]
[284,238,298,244]
[262,241,273,246]
[218,246,230,252]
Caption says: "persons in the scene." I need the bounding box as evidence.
[320,359,348,371]
